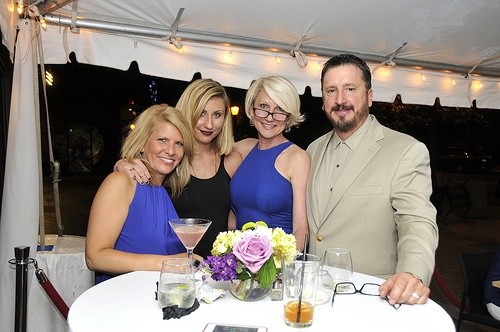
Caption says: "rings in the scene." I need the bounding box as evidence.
[130,168,135,172]
[411,292,420,298]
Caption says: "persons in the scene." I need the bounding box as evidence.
[483,244,500,321]
[305,54,439,305]
[114,79,242,268]
[232,75,311,262]
[85,104,204,286]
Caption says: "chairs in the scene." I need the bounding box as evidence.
[457,251,500,332]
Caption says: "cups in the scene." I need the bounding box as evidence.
[282,253,322,329]
[320,248,354,294]
[158,257,197,310]
[296,267,334,305]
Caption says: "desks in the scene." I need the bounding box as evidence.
[38,234,95,332]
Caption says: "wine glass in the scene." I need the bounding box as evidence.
[168,218,212,281]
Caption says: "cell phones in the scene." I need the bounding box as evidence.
[202,323,268,332]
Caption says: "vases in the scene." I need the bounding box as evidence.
[229,277,273,301]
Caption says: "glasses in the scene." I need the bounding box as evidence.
[252,99,291,122]
[331,282,402,310]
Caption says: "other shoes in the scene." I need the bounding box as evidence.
[486,303,500,320]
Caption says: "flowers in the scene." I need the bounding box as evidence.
[199,220,297,300]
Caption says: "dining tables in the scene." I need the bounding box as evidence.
[66,260,457,332]
